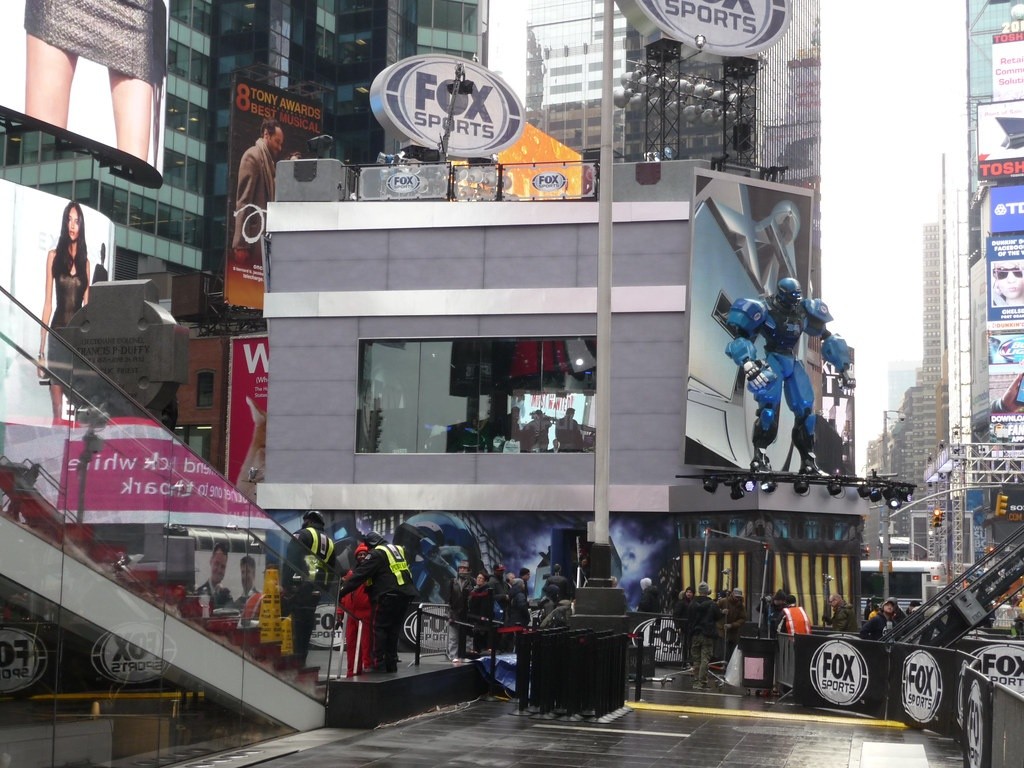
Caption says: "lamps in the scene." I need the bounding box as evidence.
[447,72,473,94]
[695,33,707,48]
[613,64,730,127]
[703,471,917,511]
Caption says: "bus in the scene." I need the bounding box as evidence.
[858,560,979,621]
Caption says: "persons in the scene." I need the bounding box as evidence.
[234,555,260,608]
[611,576,662,613]
[334,543,373,676]
[340,531,421,672]
[233,121,284,267]
[505,407,584,453]
[725,278,856,476]
[860,597,923,640]
[822,593,858,632]
[38,200,90,422]
[279,511,347,652]
[196,542,234,607]
[92,243,108,284]
[25,0,167,163]
[673,581,811,690]
[448,560,573,662]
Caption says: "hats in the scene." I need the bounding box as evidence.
[699,583,708,591]
[733,588,743,598]
[495,564,505,570]
[640,577,652,591]
[512,578,523,589]
[520,569,529,575]
[458,560,470,570]
[355,543,367,557]
[775,590,785,600]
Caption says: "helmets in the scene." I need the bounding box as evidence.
[881,597,897,613]
[304,511,325,525]
[367,532,383,545]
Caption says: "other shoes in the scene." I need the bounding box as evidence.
[452,659,472,664]
[694,682,712,691]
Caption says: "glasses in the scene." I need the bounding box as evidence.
[997,269,1022,278]
[828,600,835,603]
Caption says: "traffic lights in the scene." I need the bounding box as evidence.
[932,508,942,527]
[995,494,1008,518]
